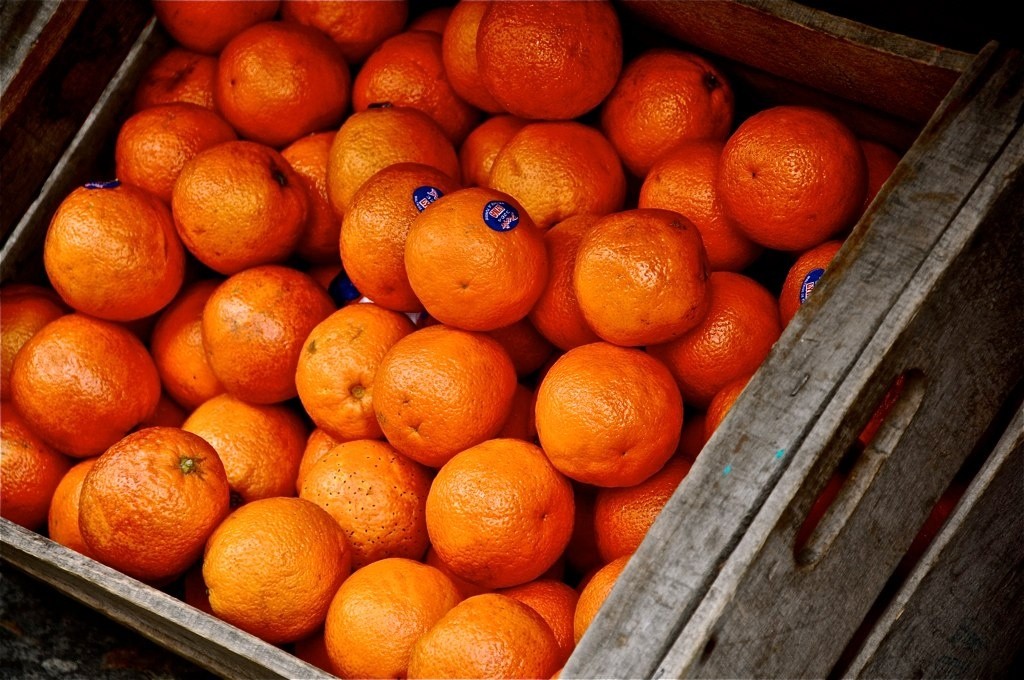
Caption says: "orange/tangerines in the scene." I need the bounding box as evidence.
[0,0,973,680]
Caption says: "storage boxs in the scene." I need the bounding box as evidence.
[0,0,1024,680]
